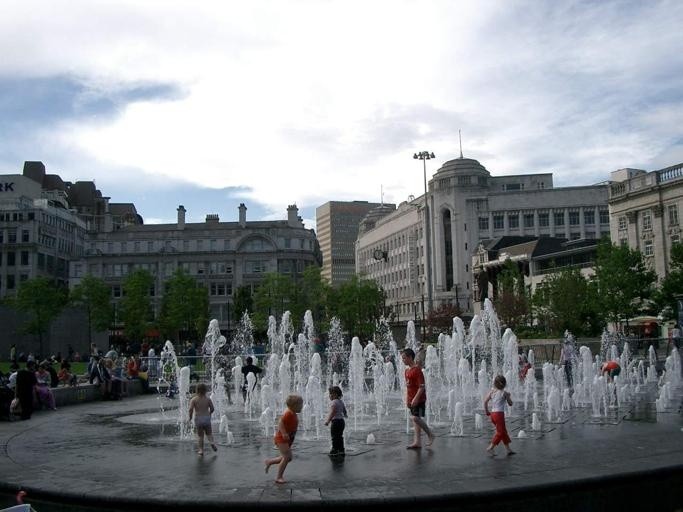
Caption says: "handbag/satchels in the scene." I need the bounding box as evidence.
[9,398,22,416]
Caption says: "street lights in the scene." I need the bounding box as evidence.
[410,148,436,335]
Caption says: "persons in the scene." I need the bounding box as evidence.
[240,356,261,406]
[324,386,347,455]
[0,338,157,420]
[188,383,216,455]
[558,339,575,386]
[671,324,680,352]
[400,348,435,450]
[600,361,620,382]
[520,355,531,378]
[483,375,518,457]
[263,395,303,482]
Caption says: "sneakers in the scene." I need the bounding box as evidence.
[328,448,345,457]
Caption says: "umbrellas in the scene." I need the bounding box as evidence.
[621,314,662,324]
